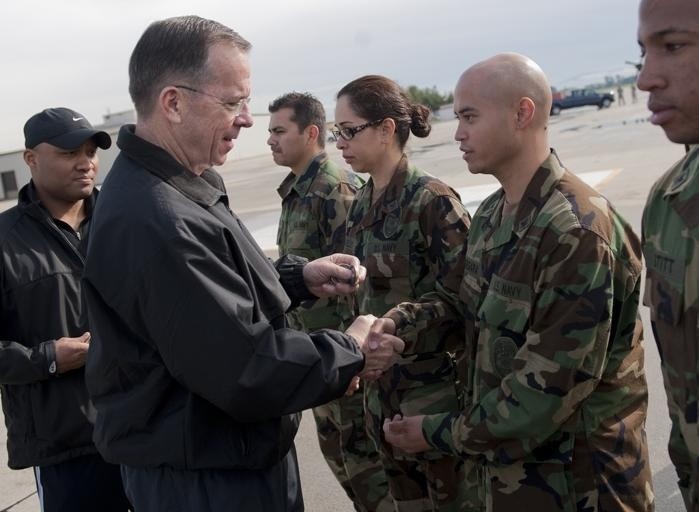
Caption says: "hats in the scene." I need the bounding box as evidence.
[23,107,112,151]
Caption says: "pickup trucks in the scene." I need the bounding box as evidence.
[552,89,614,114]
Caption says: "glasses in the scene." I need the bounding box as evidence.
[175,85,252,117]
[331,118,383,141]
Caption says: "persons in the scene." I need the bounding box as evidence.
[363,52,654,512]
[267,93,364,512]
[86,16,404,512]
[334,76,472,512]
[0,108,133,512]
[637,0,699,512]
